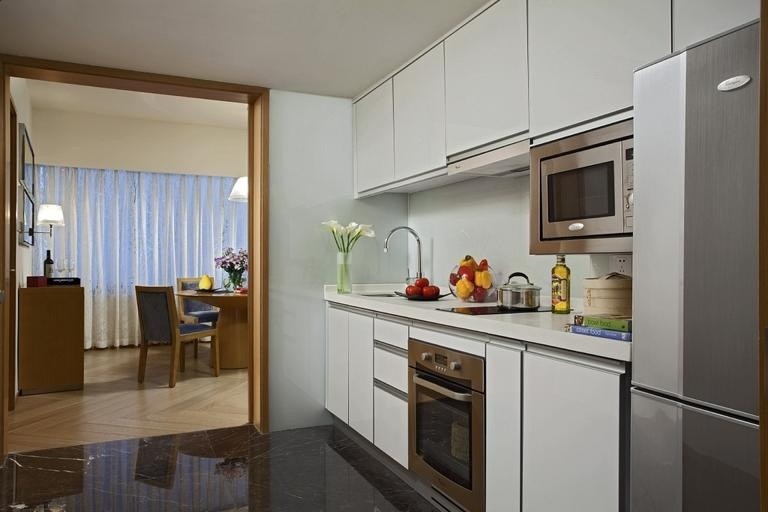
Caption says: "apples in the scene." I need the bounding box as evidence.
[406,278,439,298]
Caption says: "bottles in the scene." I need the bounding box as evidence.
[43,250,55,278]
[550,254,569,315]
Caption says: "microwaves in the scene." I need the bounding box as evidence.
[527,117,634,258]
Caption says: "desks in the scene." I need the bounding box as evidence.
[17,286,85,398]
[173,285,253,372]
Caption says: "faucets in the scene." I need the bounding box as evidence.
[383,226,422,288]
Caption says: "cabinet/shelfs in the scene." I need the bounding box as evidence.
[325,306,374,444]
[349,36,447,198]
[526,2,762,146]
[445,2,529,165]
[372,314,410,471]
[484,341,623,511]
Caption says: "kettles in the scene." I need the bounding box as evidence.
[495,271,544,310]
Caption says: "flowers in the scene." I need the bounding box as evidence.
[319,218,377,295]
[213,246,250,295]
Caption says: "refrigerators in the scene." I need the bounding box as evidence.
[624,15,760,512]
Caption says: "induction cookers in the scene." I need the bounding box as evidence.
[435,303,572,315]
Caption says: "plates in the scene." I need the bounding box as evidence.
[196,290,215,293]
[394,291,452,302]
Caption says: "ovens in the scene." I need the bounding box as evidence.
[407,368,487,511]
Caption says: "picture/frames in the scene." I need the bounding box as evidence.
[18,121,36,205]
[17,185,35,249]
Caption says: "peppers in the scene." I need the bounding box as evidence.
[450,255,492,302]
[454,307,489,315]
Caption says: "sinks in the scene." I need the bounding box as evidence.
[356,292,409,300]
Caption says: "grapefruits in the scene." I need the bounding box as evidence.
[199,274,212,291]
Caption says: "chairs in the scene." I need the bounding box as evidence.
[175,276,222,360]
[133,283,222,389]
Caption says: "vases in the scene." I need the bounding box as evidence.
[335,250,353,295]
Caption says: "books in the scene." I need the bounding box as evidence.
[573,313,631,333]
[46,278,82,285]
[562,324,632,342]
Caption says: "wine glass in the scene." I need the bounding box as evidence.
[56,257,76,279]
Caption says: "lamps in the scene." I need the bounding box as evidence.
[227,176,250,206]
[28,202,68,239]
